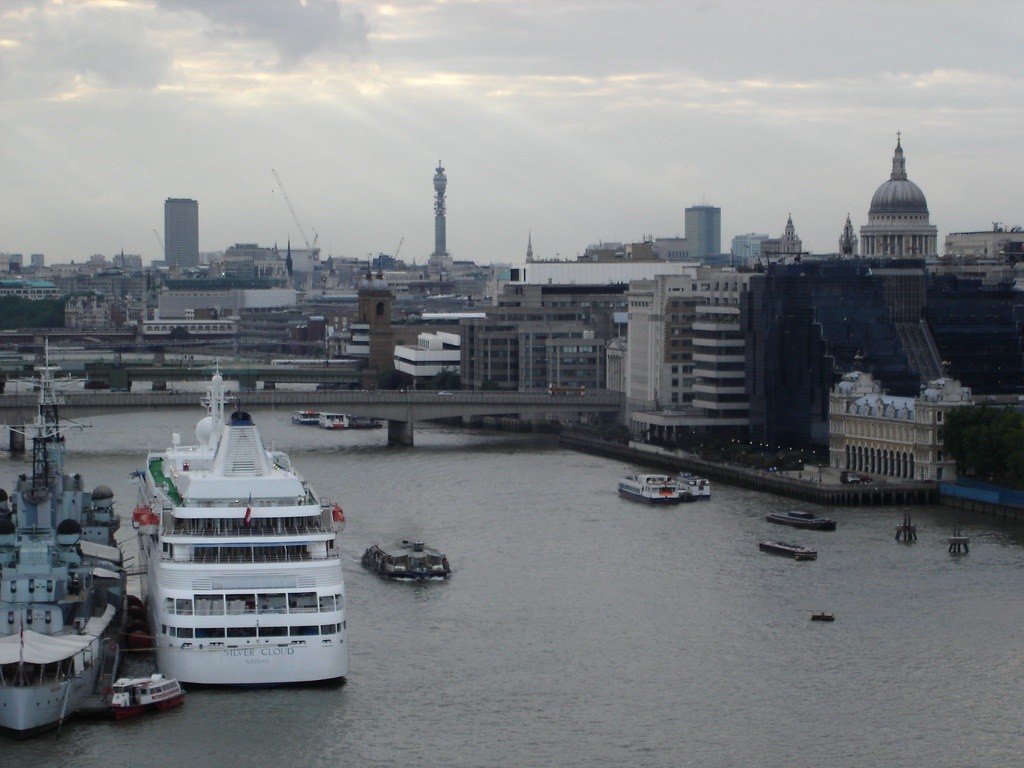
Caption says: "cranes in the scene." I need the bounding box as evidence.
[268,166,320,289]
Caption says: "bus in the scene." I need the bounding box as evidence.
[548,383,587,397]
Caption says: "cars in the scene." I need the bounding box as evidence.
[841,471,872,484]
[438,391,453,396]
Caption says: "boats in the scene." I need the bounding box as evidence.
[617,473,680,506]
[676,473,712,503]
[758,538,821,563]
[292,411,320,426]
[0,339,135,741]
[131,507,159,536]
[766,510,839,532]
[125,357,351,689]
[320,412,349,430]
[360,536,450,581]
[328,504,347,533]
[350,416,384,431]
[110,673,185,725]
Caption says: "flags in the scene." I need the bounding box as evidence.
[245,497,252,528]
